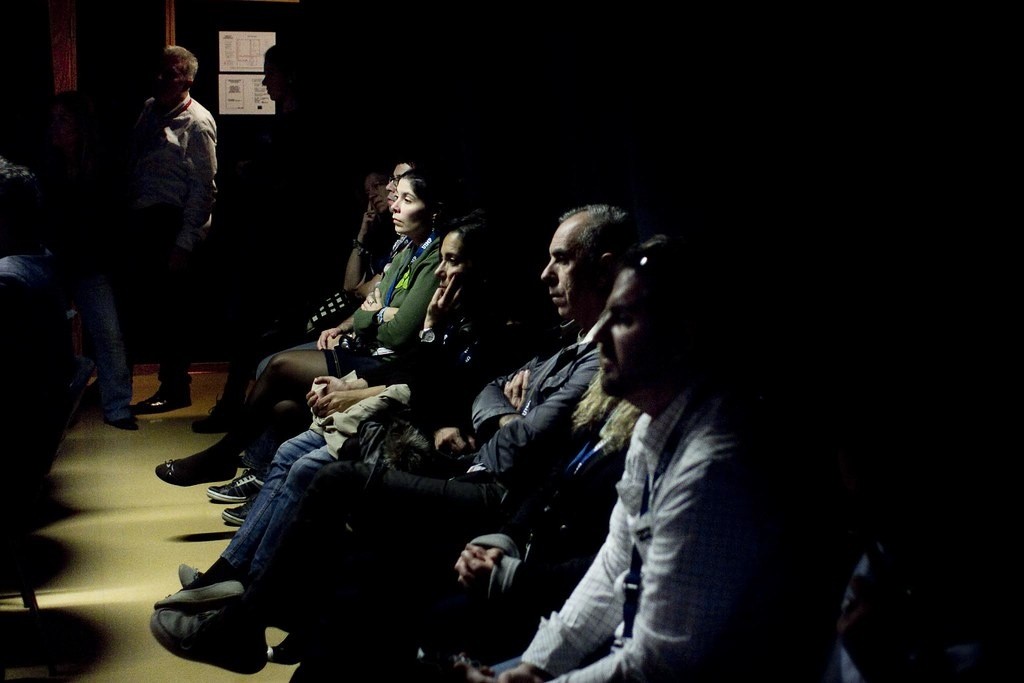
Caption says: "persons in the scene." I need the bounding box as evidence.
[157,173,764,683]
[131,43,220,413]
[233,48,341,181]
[0,77,139,594]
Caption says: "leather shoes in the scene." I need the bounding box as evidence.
[84,378,100,402]
[138,384,191,412]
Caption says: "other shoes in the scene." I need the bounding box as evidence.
[152,608,268,674]
[154,580,244,610]
[179,563,203,588]
[155,459,237,485]
[103,416,139,430]
[266,634,306,664]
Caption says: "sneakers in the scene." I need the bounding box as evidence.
[209,469,267,502]
[192,393,242,432]
[223,496,258,525]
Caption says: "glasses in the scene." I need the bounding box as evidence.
[388,177,400,188]
